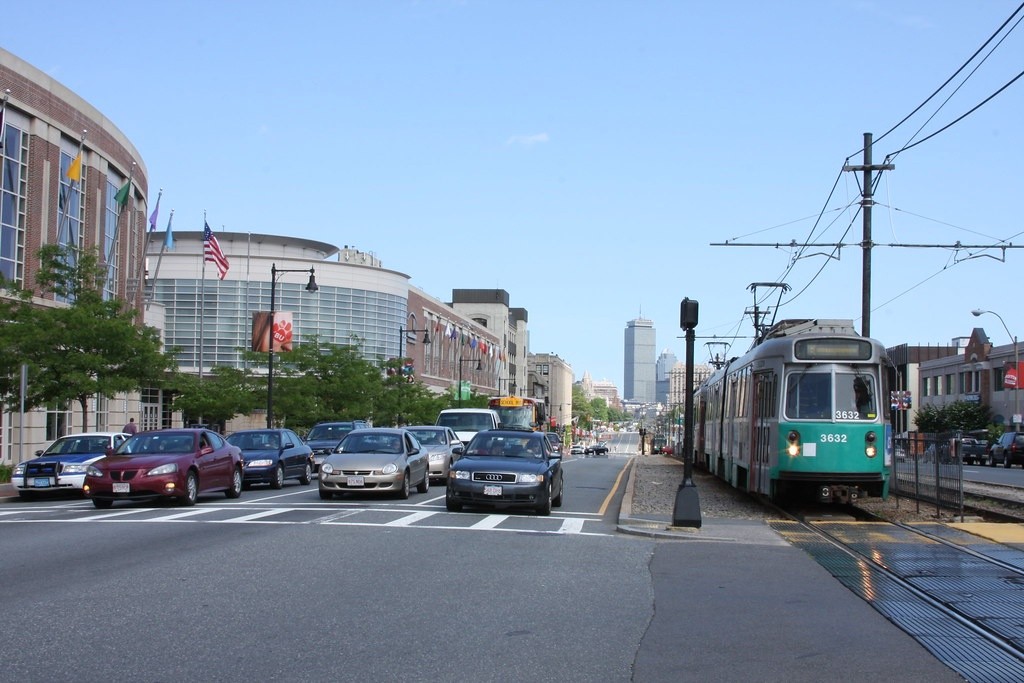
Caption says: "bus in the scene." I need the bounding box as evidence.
[486,396,546,435]
[650,434,666,454]
[669,318,893,504]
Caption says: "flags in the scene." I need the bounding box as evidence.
[435,321,507,361]
[204,219,231,280]
[114,176,132,207]
[0,108,4,142]
[65,145,84,184]
[149,197,160,230]
[163,213,174,250]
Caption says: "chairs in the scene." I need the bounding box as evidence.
[482,440,504,454]
[525,440,541,457]
[76,442,88,451]
[240,438,254,448]
[147,440,161,452]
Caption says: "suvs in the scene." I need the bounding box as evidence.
[298,421,372,473]
[988,432,1024,469]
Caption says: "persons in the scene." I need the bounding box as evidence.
[527,442,551,459]
[266,434,278,449]
[185,439,193,450]
[101,440,109,452]
[122,418,138,435]
[391,439,400,451]
[434,432,445,444]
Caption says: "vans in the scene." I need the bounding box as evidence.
[434,409,502,452]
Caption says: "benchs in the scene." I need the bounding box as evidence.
[504,444,523,456]
[417,437,433,444]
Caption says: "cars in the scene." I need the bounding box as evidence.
[318,426,465,499]
[895,442,949,465]
[570,445,583,454]
[223,429,316,489]
[11,426,246,508]
[585,444,608,455]
[446,429,563,516]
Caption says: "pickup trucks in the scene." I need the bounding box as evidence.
[949,433,989,466]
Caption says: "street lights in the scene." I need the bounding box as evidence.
[398,327,431,428]
[499,377,517,396]
[550,404,562,431]
[971,308,1019,432]
[459,356,483,408]
[267,262,319,429]
